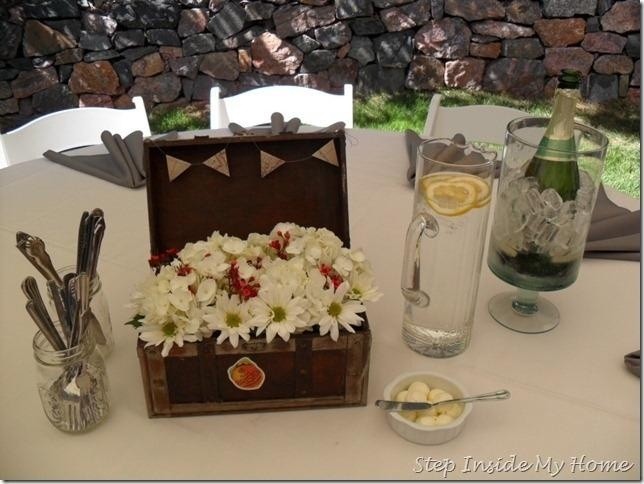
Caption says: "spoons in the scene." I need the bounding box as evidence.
[16,208,108,347]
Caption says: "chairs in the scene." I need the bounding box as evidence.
[209,83,352,130]
[0,97,151,167]
[420,95,584,162]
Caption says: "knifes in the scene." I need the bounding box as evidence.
[375,390,510,411]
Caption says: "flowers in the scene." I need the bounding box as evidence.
[125,220,385,358]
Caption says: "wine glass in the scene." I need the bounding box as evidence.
[486,115,611,335]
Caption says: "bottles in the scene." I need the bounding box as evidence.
[510,67,584,278]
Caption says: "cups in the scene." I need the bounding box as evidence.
[398,135,499,359]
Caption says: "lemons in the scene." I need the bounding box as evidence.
[421,174,492,216]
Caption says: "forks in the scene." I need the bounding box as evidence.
[14,275,109,432]
